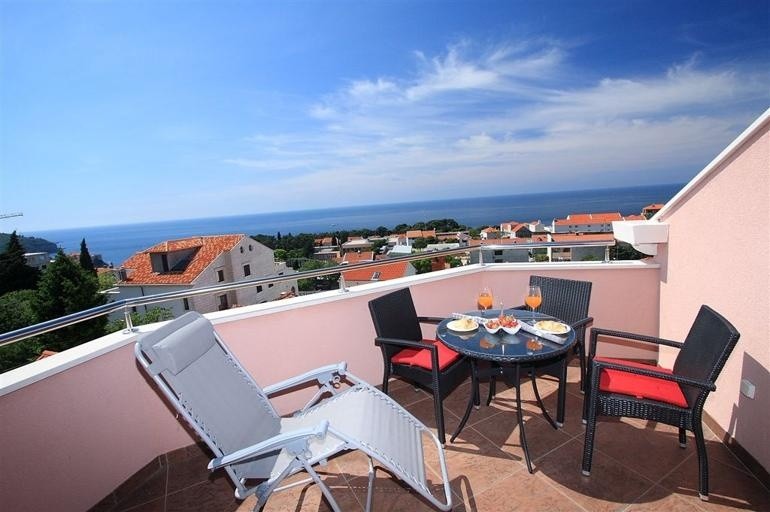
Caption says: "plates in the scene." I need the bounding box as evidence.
[446,330,478,337]
[533,320,573,335]
[446,320,478,333]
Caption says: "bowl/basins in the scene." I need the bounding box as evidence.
[488,334,520,345]
[483,316,520,334]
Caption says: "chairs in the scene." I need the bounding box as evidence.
[134,310,453,512]
[368,287,482,448]
[580,305,741,501]
[492,275,592,427]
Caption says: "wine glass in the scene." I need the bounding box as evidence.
[526,284,542,325]
[477,287,491,320]
[479,332,494,349]
[527,326,543,351]
